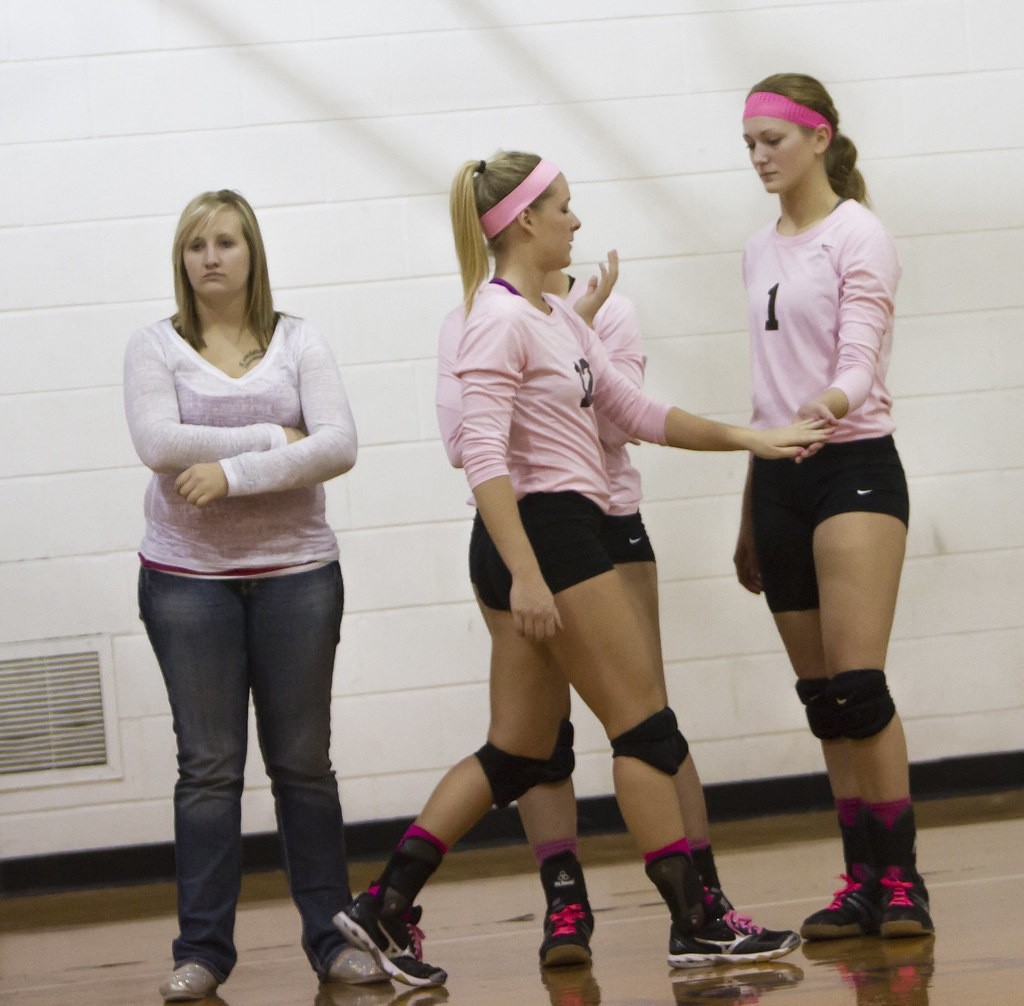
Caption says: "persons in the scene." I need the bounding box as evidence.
[333,151,838,987]
[732,74,936,938]
[122,190,393,999]
[434,265,732,969]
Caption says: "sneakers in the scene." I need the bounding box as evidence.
[802,941,890,986]
[882,939,935,994]
[800,876,887,940]
[387,985,449,1006]
[539,893,594,967]
[539,968,601,1006]
[668,908,801,968]
[879,866,935,937]
[332,881,447,986]
[668,962,804,1005]
[702,883,734,913]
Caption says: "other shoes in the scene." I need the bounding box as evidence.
[327,946,391,985]
[159,963,219,1000]
[313,984,395,1006]
